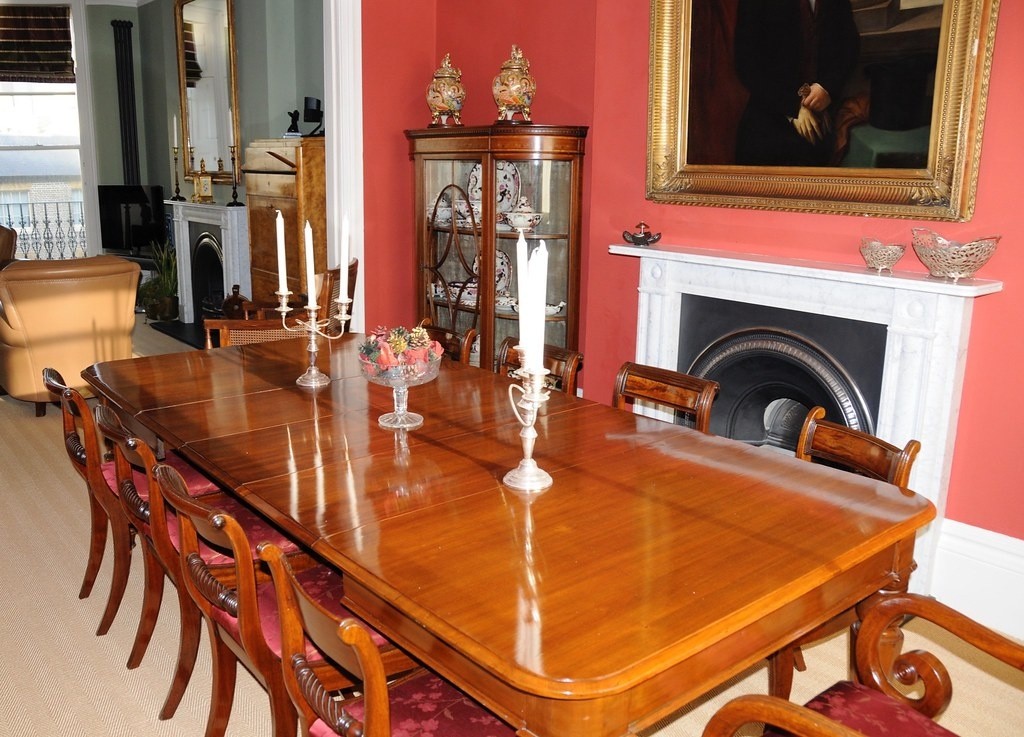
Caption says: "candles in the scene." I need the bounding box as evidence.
[274,208,289,294]
[339,217,349,300]
[173,114,178,147]
[303,221,316,306]
[516,231,549,373]
[228,107,235,146]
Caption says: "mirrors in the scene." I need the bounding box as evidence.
[174,0,241,185]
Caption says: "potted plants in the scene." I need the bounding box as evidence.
[138,242,180,321]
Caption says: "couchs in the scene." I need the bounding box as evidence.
[0,224,141,416]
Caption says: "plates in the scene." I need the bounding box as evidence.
[467,159,521,220]
[473,247,511,295]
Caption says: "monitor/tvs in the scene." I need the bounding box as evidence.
[97,185,167,251]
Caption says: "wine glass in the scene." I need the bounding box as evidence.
[360,355,441,428]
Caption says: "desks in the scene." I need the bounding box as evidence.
[81,334,936,737]
[104,250,175,273]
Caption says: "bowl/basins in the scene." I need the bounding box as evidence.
[429,200,544,232]
[436,280,561,315]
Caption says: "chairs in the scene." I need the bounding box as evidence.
[43,259,1023,737]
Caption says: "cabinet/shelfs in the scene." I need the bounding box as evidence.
[245,137,325,318]
[404,123,588,393]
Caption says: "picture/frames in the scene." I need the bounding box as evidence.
[645,0,1001,224]
[192,174,212,199]
